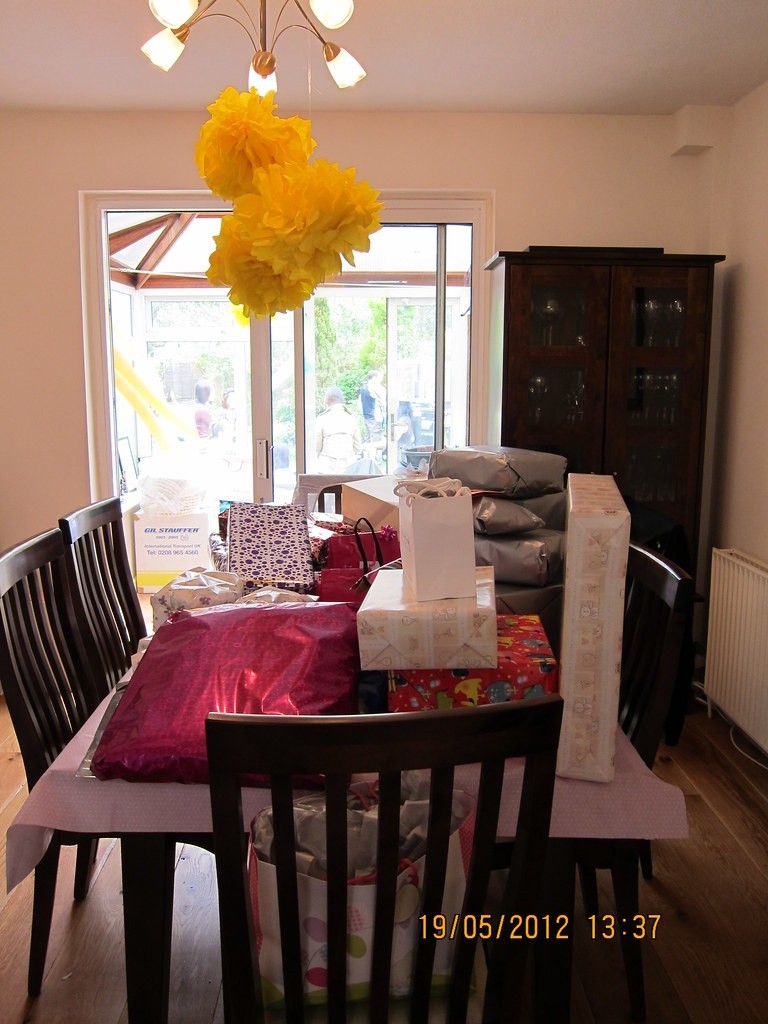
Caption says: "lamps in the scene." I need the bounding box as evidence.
[147,0,366,106]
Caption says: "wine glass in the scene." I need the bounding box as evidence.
[526,371,583,428]
[640,290,683,350]
[530,285,569,348]
[626,371,681,426]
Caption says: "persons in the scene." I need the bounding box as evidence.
[172,357,443,495]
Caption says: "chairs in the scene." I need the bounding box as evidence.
[205,694,569,1023]
[59,496,148,865]
[491,544,695,1024]
[298,474,388,514]
[0,527,248,999]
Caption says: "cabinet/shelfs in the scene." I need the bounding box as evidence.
[482,245,727,691]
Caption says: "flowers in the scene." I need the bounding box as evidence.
[195,87,385,320]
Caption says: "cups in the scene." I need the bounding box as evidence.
[632,455,677,504]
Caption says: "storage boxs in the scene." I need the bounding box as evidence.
[386,613,560,720]
[552,472,632,782]
[340,477,399,537]
[355,567,498,668]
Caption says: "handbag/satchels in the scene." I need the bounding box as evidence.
[246,775,475,1010]
[393,479,484,601]
[318,518,402,603]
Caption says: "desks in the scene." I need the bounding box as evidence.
[6,666,691,1024]
